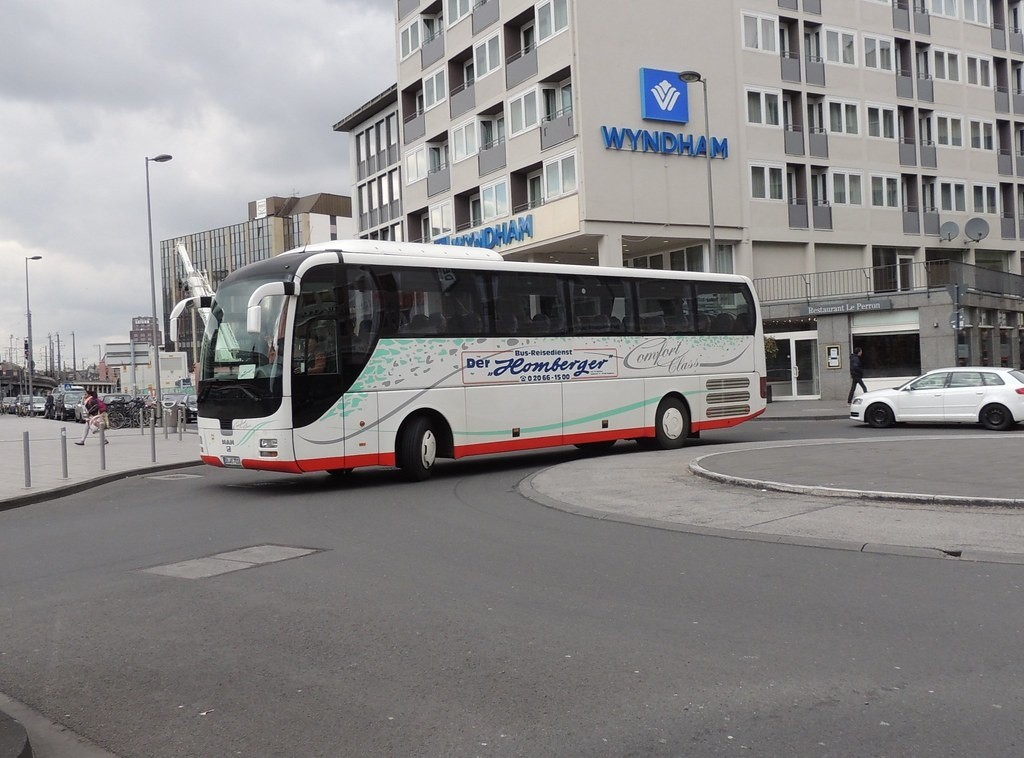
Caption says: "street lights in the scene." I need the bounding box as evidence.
[145,154,174,429]
[25,255,43,415]
[678,70,719,274]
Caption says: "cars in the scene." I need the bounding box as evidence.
[848,365,1024,431]
[178,394,197,424]
[0,383,176,430]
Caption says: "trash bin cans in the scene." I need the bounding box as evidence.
[767,385,772,403]
[161,400,177,433]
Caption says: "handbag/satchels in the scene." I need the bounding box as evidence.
[89,415,105,434]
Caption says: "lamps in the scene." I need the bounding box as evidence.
[933,322,938,327]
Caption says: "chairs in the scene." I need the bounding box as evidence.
[359,312,749,331]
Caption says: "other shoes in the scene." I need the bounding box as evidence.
[75,441,84,445]
[104,440,109,445]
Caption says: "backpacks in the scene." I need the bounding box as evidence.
[96,399,107,411]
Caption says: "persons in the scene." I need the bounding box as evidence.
[74,391,109,445]
[847,347,867,406]
[294,335,326,374]
[45,391,54,419]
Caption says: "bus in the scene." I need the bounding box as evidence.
[169,240,766,483]
